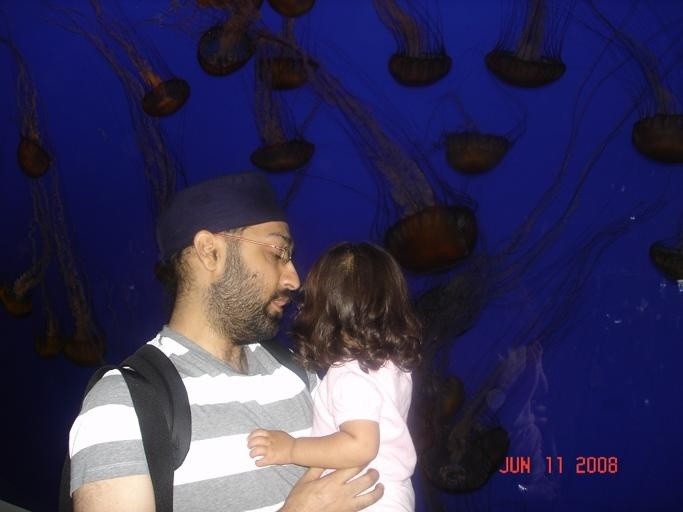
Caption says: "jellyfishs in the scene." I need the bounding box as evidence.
[3,0,682,497]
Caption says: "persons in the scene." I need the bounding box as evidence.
[248,240,424,512]
[57,173,383,512]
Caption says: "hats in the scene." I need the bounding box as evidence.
[156,172,288,256]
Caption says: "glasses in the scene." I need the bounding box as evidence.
[222,233,292,265]
